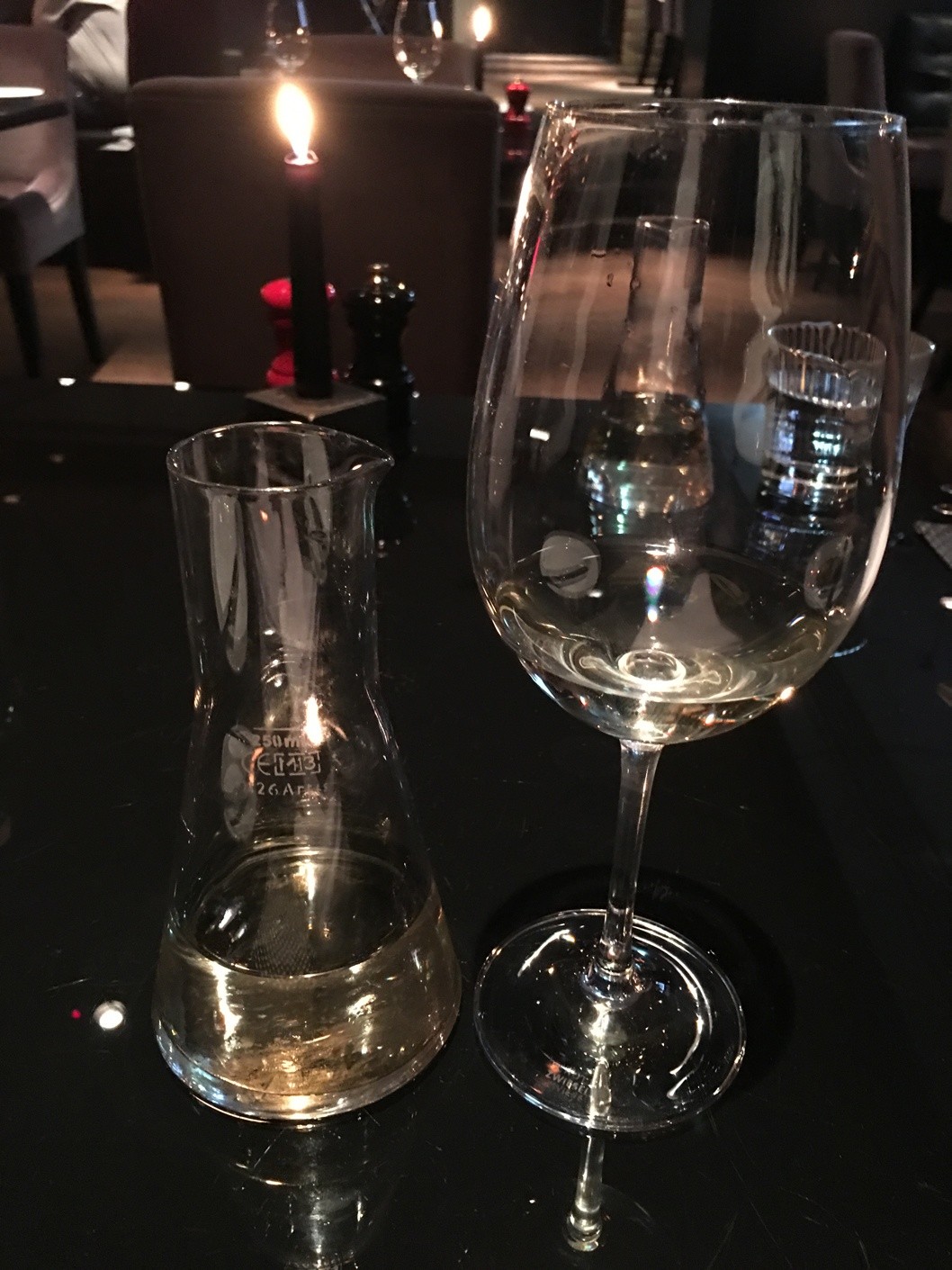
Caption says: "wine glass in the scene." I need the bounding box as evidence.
[476,95,908,1132]
[266,1,314,77]
[389,1,443,87]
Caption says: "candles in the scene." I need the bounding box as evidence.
[278,84,333,400]
[471,4,491,88]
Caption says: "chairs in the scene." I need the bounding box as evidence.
[1,22,951,428]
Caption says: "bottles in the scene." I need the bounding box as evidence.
[149,423,464,1126]
[578,212,717,516]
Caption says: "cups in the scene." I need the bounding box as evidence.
[754,321,888,538]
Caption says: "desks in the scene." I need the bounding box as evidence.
[0,379,952,1270]
[498,113,539,175]
